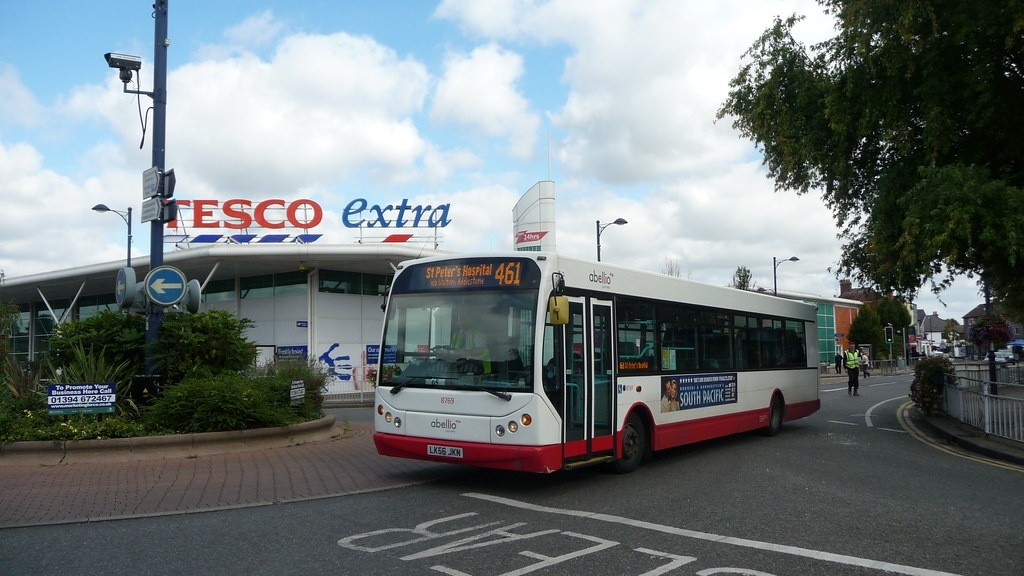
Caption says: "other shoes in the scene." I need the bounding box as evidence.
[848,388,851,395]
[868,373,870,378]
[853,390,860,396]
[864,375,867,378]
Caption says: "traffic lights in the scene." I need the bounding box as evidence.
[885,327,893,344]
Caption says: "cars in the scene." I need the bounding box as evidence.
[1014,354,1019,364]
[998,350,1016,365]
[983,352,1007,368]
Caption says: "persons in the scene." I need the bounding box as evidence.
[834,342,871,396]
[450,311,492,374]
[661,379,679,413]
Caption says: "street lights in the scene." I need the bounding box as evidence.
[596,218,628,263]
[92,204,133,268]
[772,256,800,298]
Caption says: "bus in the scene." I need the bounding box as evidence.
[372,252,821,475]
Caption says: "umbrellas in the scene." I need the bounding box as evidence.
[1006,339,1023,346]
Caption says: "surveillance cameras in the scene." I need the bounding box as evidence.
[104,53,141,70]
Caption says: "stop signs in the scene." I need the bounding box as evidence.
[916,334,922,341]
